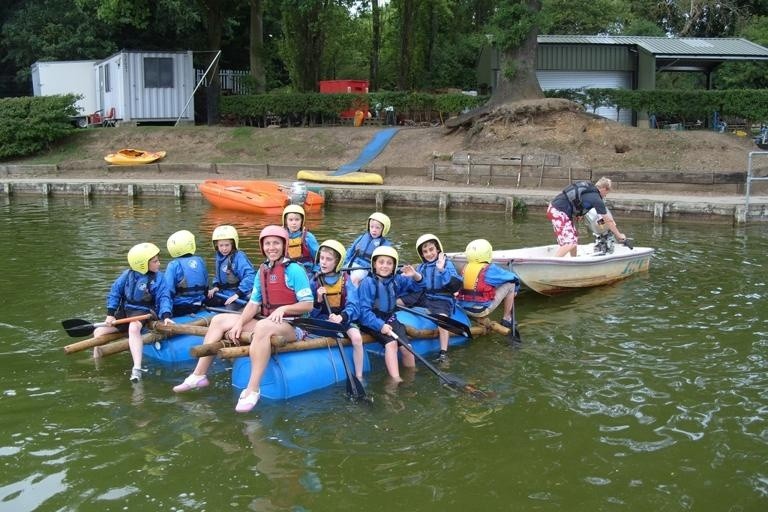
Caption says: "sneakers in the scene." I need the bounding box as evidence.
[172,374,210,392]
[235,388,261,414]
[129,366,149,383]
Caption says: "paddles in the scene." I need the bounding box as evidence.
[395,306,472,338]
[203,304,350,339]
[61,309,158,338]
[506,258,520,341]
[390,332,466,393]
[317,276,364,396]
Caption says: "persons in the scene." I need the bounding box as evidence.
[309,239,368,388]
[358,246,427,378]
[416,234,464,362]
[456,239,520,338]
[547,176,626,256]
[342,212,392,286]
[164,230,209,318]
[93,243,176,381]
[206,225,256,310]
[173,226,313,413]
[282,205,319,279]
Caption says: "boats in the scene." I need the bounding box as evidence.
[197,176,325,217]
[64,285,512,409]
[439,239,655,299]
[105,146,168,166]
[296,169,383,187]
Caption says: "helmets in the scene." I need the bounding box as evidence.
[314,240,347,273]
[259,225,289,258]
[127,242,161,275]
[465,239,493,264]
[167,230,196,258]
[282,204,305,229]
[367,212,391,237]
[370,245,399,275]
[415,233,444,264]
[211,224,239,251]
